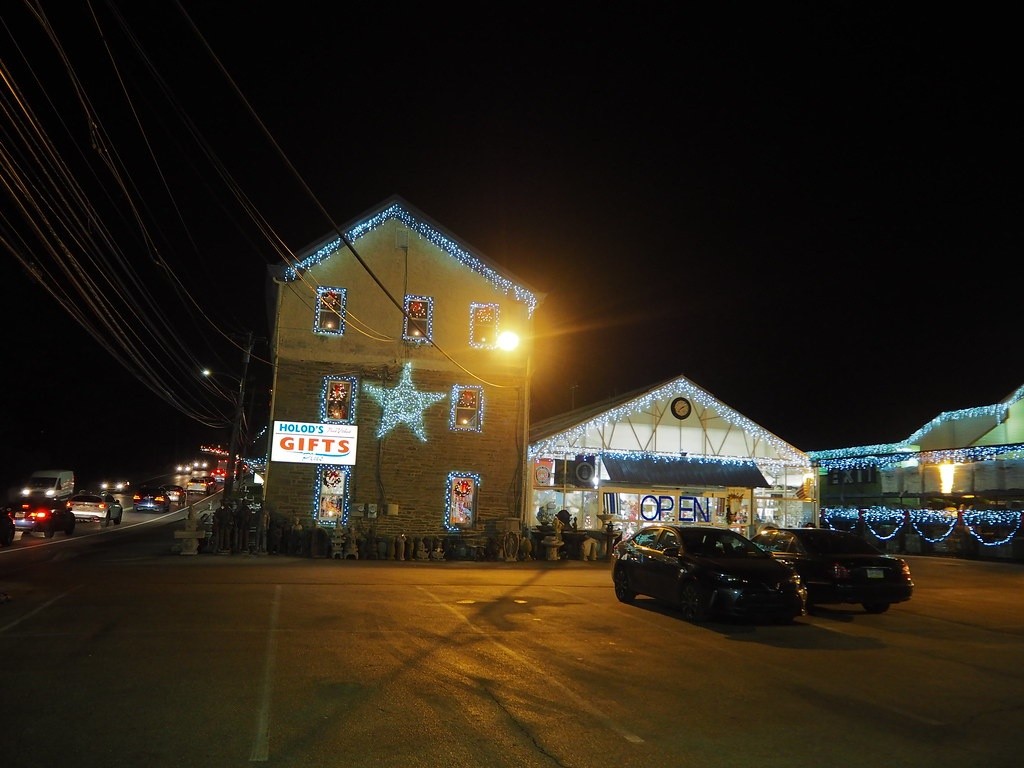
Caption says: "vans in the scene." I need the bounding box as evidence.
[22,470,75,502]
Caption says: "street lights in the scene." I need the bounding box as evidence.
[202,369,242,387]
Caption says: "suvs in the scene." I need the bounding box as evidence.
[133,485,170,515]
[187,477,211,497]
[65,489,123,528]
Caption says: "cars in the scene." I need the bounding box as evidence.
[0,510,16,549]
[158,484,187,504]
[177,445,268,475]
[4,498,76,539]
[100,477,130,493]
[209,468,227,483]
[201,476,218,491]
[747,528,915,613]
[608,525,810,625]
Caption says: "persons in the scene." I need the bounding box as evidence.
[209,495,253,555]
